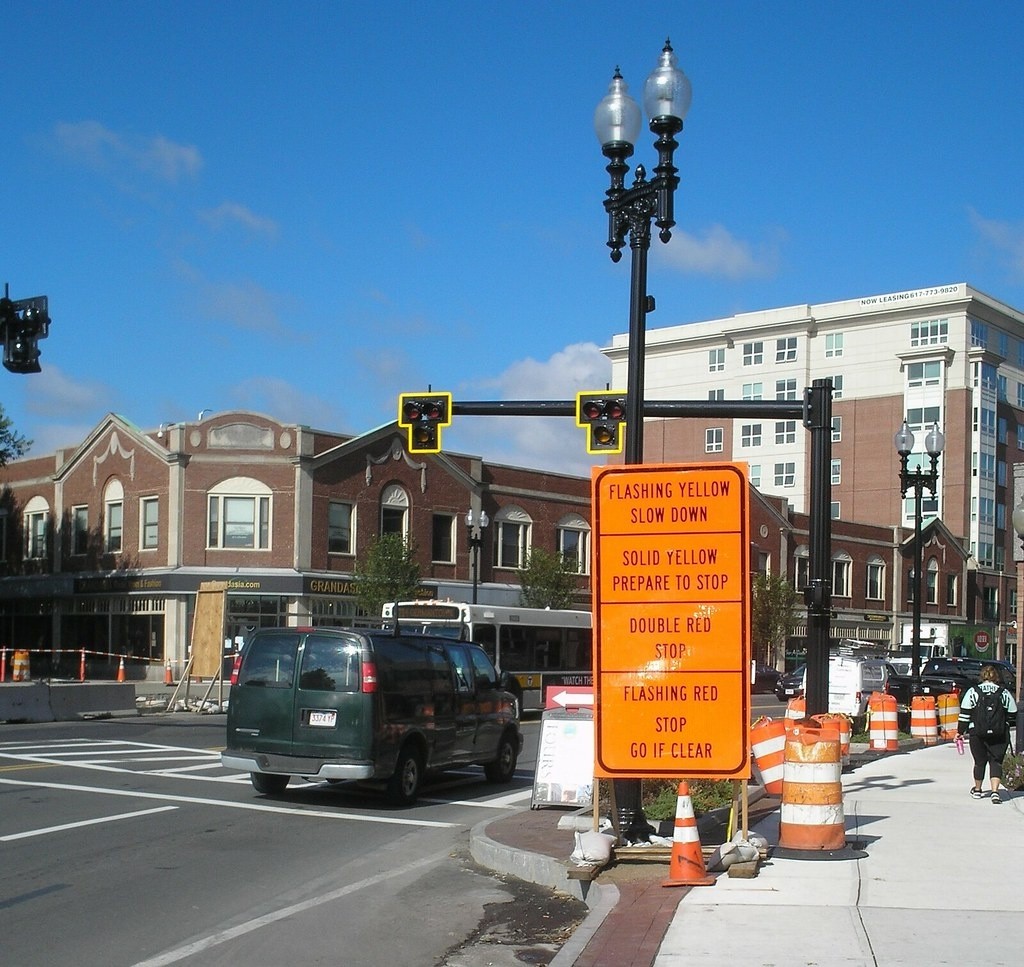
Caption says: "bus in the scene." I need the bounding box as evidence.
[382,600,593,713]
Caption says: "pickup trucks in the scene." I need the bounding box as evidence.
[885,662,961,709]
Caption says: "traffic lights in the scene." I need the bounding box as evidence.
[397,392,452,453]
[575,391,628,455]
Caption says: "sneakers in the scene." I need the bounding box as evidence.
[990,792,1002,804]
[969,787,981,798]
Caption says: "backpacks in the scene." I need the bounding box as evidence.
[970,685,1009,739]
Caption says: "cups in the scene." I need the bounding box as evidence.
[956,738,964,755]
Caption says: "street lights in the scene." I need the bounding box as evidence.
[895,416,946,735]
[595,39,696,852]
[465,509,489,608]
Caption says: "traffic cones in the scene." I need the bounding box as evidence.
[659,781,717,887]
[166,658,176,686]
[116,657,127,683]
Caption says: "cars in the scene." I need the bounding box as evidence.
[749,665,784,694]
[777,662,809,702]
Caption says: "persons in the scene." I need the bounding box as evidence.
[953,665,1018,804]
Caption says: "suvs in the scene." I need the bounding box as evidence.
[220,625,524,808]
[802,654,901,734]
[923,657,1017,728]
